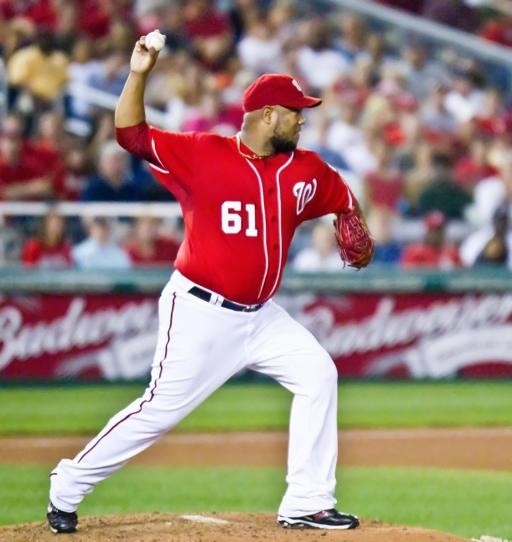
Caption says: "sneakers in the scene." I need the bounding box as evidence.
[47,501,79,535]
[276,507,360,531]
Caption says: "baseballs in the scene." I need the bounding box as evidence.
[146,32,165,51]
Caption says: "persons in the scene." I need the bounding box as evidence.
[46,30,374,534]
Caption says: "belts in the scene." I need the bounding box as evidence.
[188,285,264,313]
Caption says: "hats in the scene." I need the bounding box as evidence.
[240,72,322,114]
